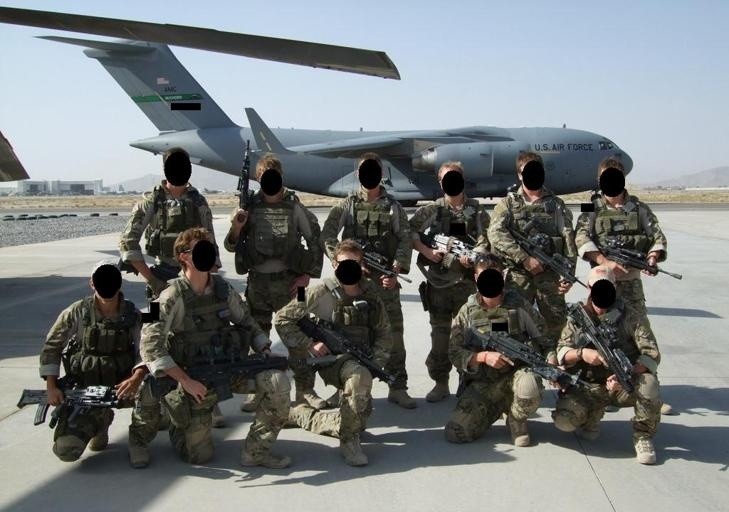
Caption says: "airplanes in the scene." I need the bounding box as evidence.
[30,32,633,206]
[0,6,401,186]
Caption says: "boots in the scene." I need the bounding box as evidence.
[427,380,452,403]
[661,402,673,415]
[211,404,226,429]
[578,420,602,443]
[129,433,151,469]
[158,415,172,431]
[633,429,657,465]
[326,390,341,407]
[241,394,257,412]
[339,432,370,467]
[506,412,530,448]
[296,388,327,409]
[240,446,292,469]
[388,387,419,410]
[89,408,114,452]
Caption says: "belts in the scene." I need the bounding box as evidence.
[173,357,247,366]
[250,270,299,284]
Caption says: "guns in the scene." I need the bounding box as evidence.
[357,243,413,290]
[567,300,638,394]
[235,139,253,224]
[464,325,590,394]
[297,316,401,390]
[418,233,486,271]
[502,225,591,292]
[596,243,683,281]
[145,356,291,400]
[150,265,182,282]
[17,387,137,428]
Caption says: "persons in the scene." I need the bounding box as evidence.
[274,239,394,466]
[410,161,490,403]
[119,147,222,311]
[444,254,563,445]
[556,266,662,464]
[321,153,417,408]
[487,151,577,340]
[138,228,293,470]
[223,151,325,412]
[575,158,667,324]
[40,261,161,470]
